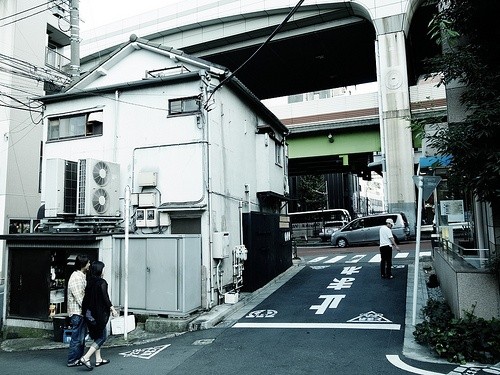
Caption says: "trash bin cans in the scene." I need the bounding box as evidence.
[52,317,69,342]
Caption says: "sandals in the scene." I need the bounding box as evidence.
[96,360,110,366]
[79,356,93,370]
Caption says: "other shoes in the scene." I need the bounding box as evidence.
[67,360,84,366]
[380,274,384,278]
[385,275,393,280]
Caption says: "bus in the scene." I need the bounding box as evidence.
[288,209,351,239]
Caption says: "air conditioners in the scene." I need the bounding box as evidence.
[45,159,121,217]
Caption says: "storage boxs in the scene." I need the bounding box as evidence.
[110,315,136,335]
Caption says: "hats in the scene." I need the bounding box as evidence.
[386,219,395,226]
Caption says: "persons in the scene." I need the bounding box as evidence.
[66,254,90,366]
[82,252,119,369]
[379,219,401,279]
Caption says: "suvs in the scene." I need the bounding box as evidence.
[330,212,411,246]
[318,220,349,239]
